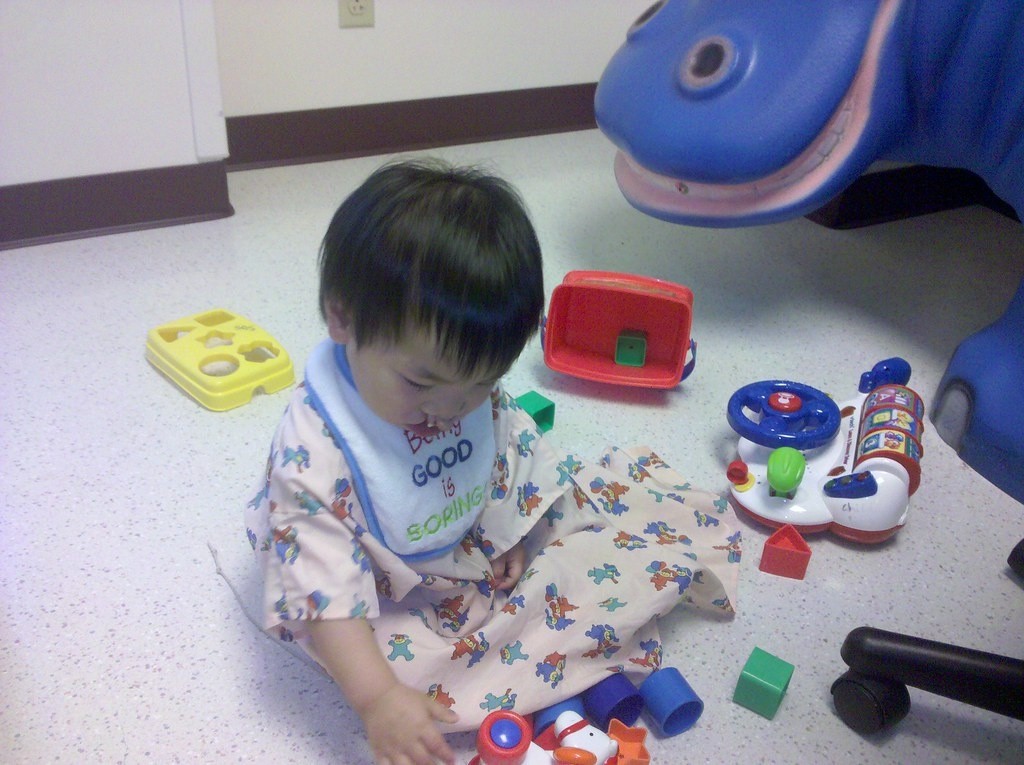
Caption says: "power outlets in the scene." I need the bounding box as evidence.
[338,0,375,29]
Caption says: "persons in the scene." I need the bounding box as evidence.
[244,158,740,765]
